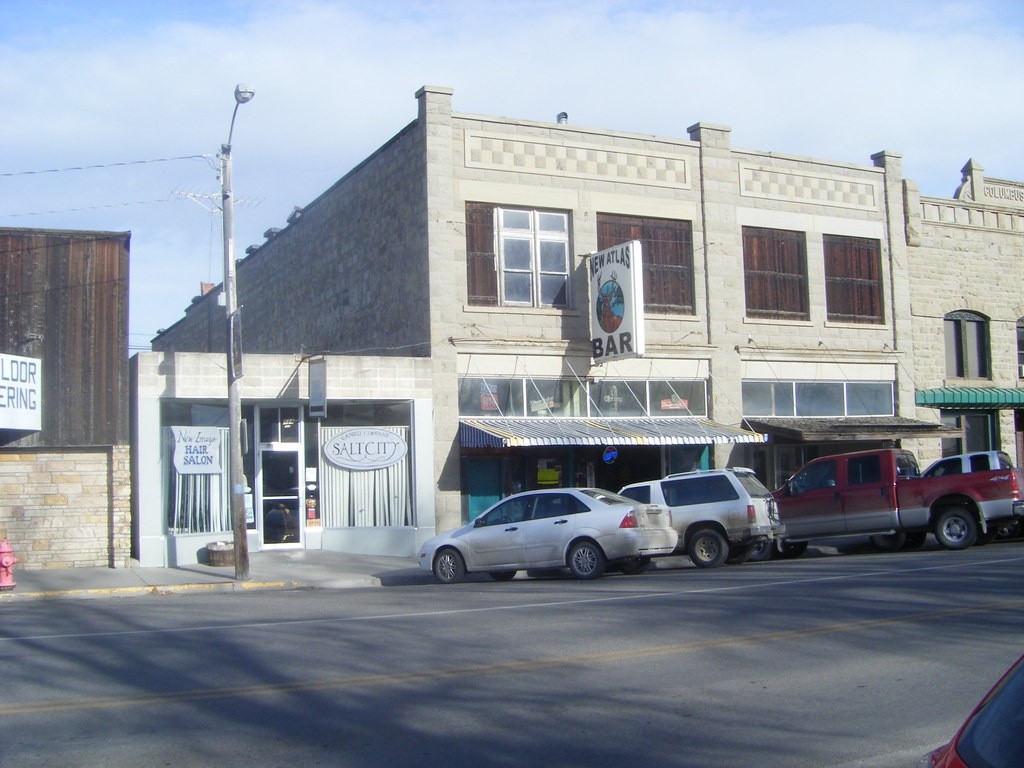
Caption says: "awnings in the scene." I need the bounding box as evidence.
[915,387,1024,409]
[459,418,768,448]
[742,416,963,441]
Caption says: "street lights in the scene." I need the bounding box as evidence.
[216,83,259,582]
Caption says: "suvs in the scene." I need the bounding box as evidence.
[617,466,788,569]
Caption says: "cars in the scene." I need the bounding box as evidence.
[418,486,680,585]
[909,655,1024,767]
[920,450,1011,478]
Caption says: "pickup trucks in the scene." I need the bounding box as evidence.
[768,447,1024,561]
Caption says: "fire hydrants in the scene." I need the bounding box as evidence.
[0,538,19,593]
